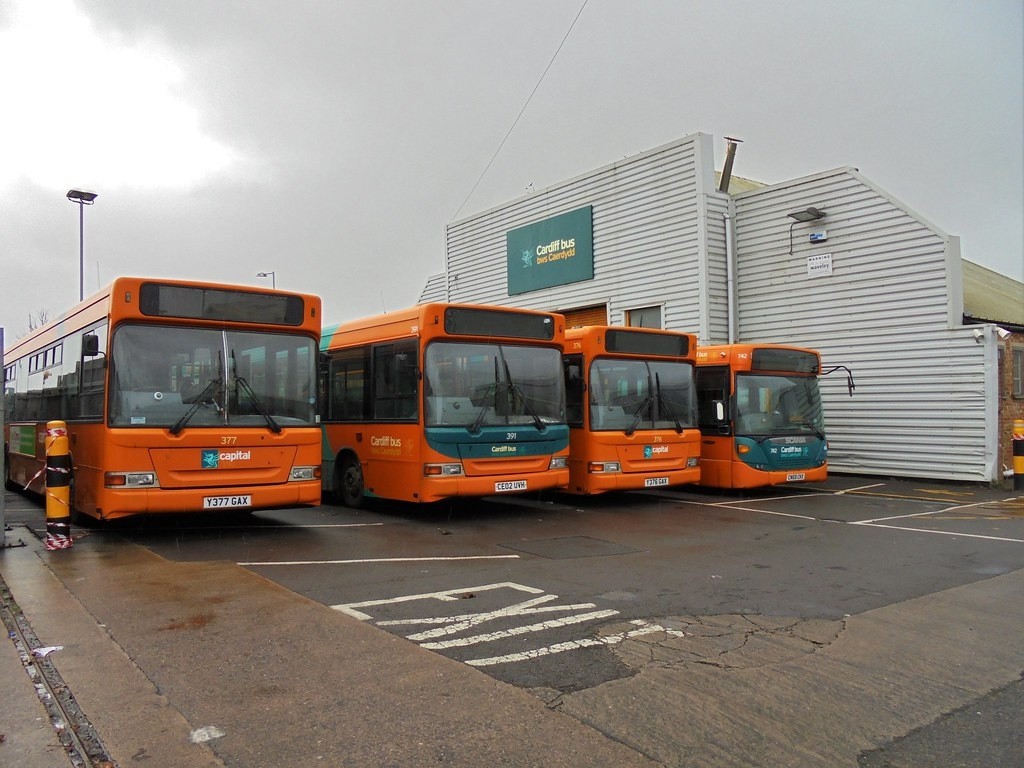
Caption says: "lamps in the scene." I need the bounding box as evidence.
[971,329,985,344]
[787,207,826,223]
[992,326,1011,340]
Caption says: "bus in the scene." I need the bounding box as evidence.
[1,277,326,522]
[333,362,462,403]
[224,303,570,508]
[472,325,703,494]
[690,343,858,491]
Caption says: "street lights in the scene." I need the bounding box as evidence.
[67,189,98,302]
[258,271,276,288]
